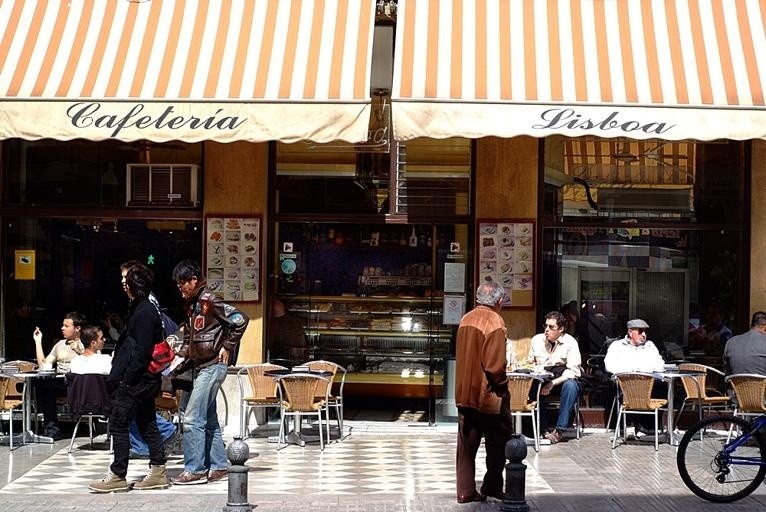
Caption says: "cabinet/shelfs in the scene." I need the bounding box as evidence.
[271,294,453,395]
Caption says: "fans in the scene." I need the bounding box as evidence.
[118,142,184,153]
[560,137,688,163]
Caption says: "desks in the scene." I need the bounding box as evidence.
[262,370,334,446]
[649,370,707,446]
[505,371,554,434]
[507,369,552,447]
[0,369,56,445]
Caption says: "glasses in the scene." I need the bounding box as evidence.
[637,329,648,333]
[543,323,556,328]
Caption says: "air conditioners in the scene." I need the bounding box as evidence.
[125,161,201,208]
[595,187,690,213]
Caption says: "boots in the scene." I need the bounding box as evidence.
[129,465,169,489]
[88,472,129,493]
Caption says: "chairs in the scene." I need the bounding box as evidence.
[724,373,766,445]
[611,372,672,450]
[586,355,626,438]
[544,365,585,439]
[0,361,38,450]
[274,373,330,451]
[67,411,114,454]
[155,387,183,453]
[505,373,544,452]
[301,361,346,440]
[668,363,735,444]
[237,363,289,441]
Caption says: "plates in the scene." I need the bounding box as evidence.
[41,369,55,372]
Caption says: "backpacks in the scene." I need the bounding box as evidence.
[151,299,179,340]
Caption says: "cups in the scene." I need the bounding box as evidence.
[40,363,52,369]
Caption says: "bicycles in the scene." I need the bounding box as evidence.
[676,414,766,503]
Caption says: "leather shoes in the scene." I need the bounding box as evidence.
[457,489,486,503]
[480,485,505,500]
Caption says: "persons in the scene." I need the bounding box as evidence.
[579,302,614,354]
[722,310,766,447]
[526,311,592,444]
[455,279,512,503]
[88,262,249,492]
[696,304,733,354]
[603,319,672,439]
[33,312,131,440]
[559,300,580,342]
[267,300,307,369]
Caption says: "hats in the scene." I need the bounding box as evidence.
[627,319,649,328]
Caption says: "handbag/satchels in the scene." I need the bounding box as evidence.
[147,341,175,374]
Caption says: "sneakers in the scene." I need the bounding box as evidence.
[545,430,562,443]
[171,471,208,485]
[209,469,227,481]
